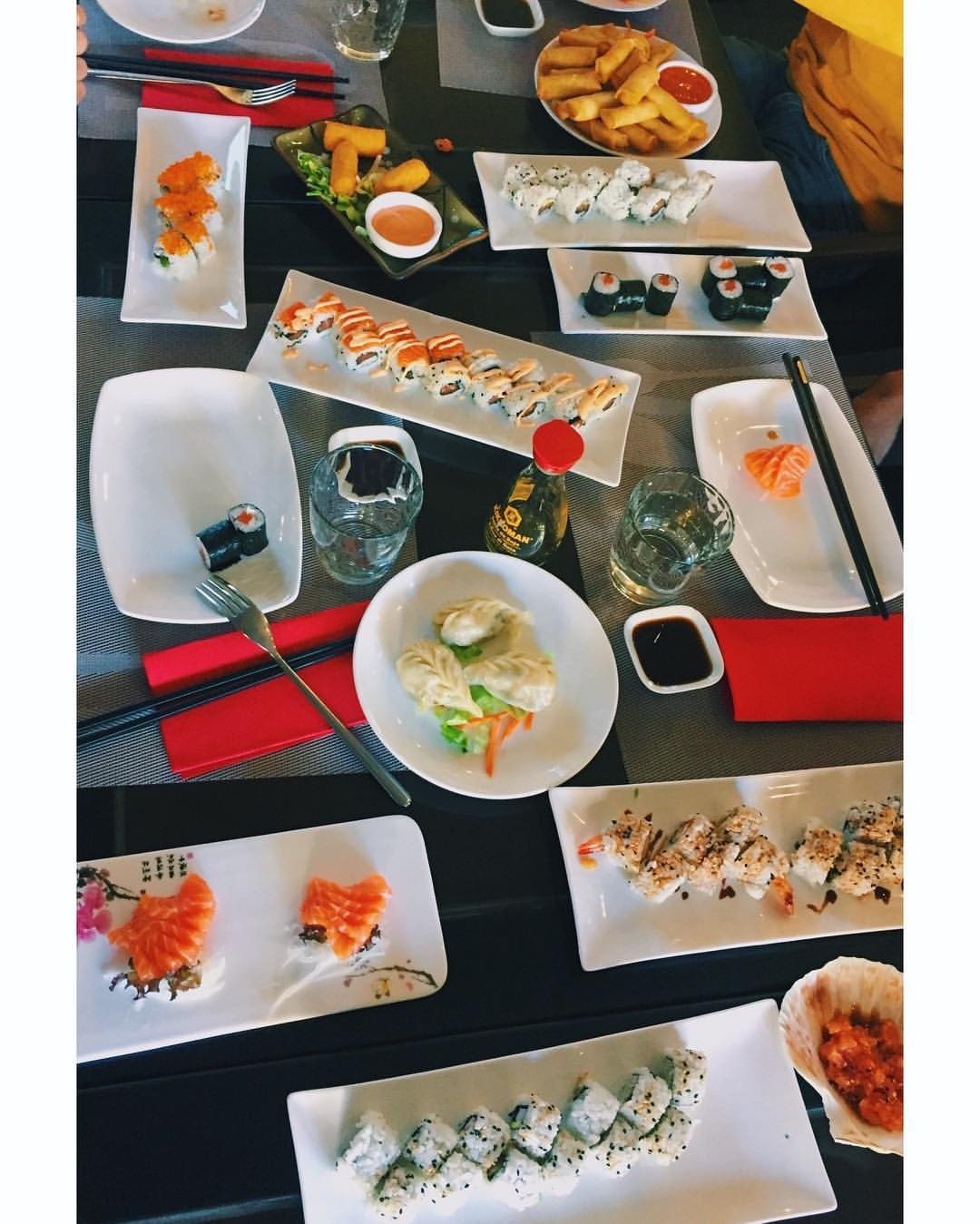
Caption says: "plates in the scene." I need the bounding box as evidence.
[86,362,306,626]
[270,98,488,283]
[287,995,839,1223]
[471,148,815,258]
[656,60,718,114]
[352,549,620,801]
[243,270,642,490]
[533,24,724,159]
[76,809,450,1070]
[118,103,256,330]
[547,759,903,977]
[545,244,830,345]
[475,0,547,38]
[95,0,267,46]
[688,371,903,617]
[623,605,725,697]
[327,425,425,504]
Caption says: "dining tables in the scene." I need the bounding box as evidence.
[79,0,902,1223]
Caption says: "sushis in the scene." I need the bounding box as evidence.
[149,144,226,284]
[272,294,631,445]
[596,792,903,909]
[574,258,793,333]
[329,1049,708,1215]
[499,152,716,234]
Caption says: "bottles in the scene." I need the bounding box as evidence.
[578,0,669,14]
[481,418,590,577]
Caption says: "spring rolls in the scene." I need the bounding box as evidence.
[536,19,706,151]
[316,116,431,207]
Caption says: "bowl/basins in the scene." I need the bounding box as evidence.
[365,190,444,260]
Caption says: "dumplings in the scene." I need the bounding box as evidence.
[405,595,556,726]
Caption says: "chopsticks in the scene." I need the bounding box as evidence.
[782,353,894,622]
[81,51,350,101]
[77,625,366,760]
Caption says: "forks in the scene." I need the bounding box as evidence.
[196,569,413,808]
[87,67,297,108]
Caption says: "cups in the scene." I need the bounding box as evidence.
[307,441,426,586]
[328,0,409,60]
[610,469,739,606]
